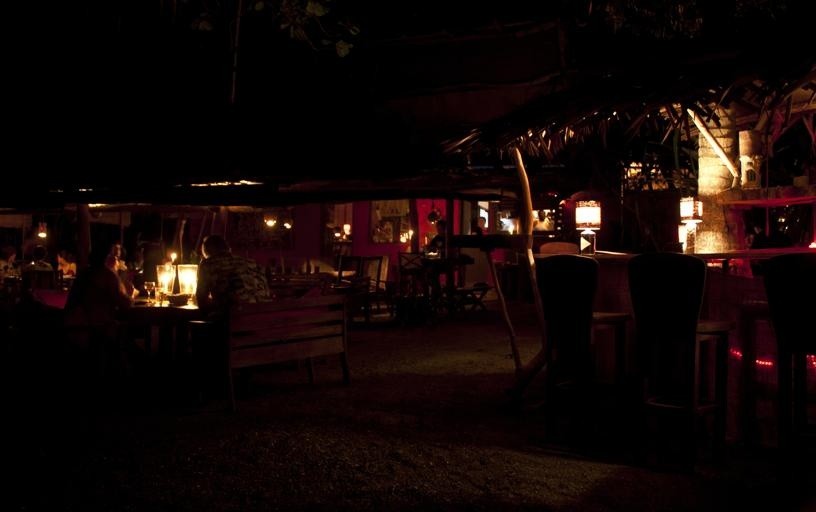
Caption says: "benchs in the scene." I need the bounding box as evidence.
[217,296,355,408]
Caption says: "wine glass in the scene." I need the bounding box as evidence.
[143,281,155,304]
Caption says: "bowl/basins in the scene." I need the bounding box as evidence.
[166,293,192,308]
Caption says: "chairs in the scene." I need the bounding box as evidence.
[762,250,816,448]
[623,251,732,434]
[337,252,491,321]
[534,255,632,396]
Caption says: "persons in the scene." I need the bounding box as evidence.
[533,210,554,232]
[60,253,139,351]
[748,204,797,302]
[10,246,53,279]
[174,234,245,349]
[108,240,129,273]
[478,216,490,238]
[423,218,465,300]
[55,249,76,278]
[0,243,15,288]
